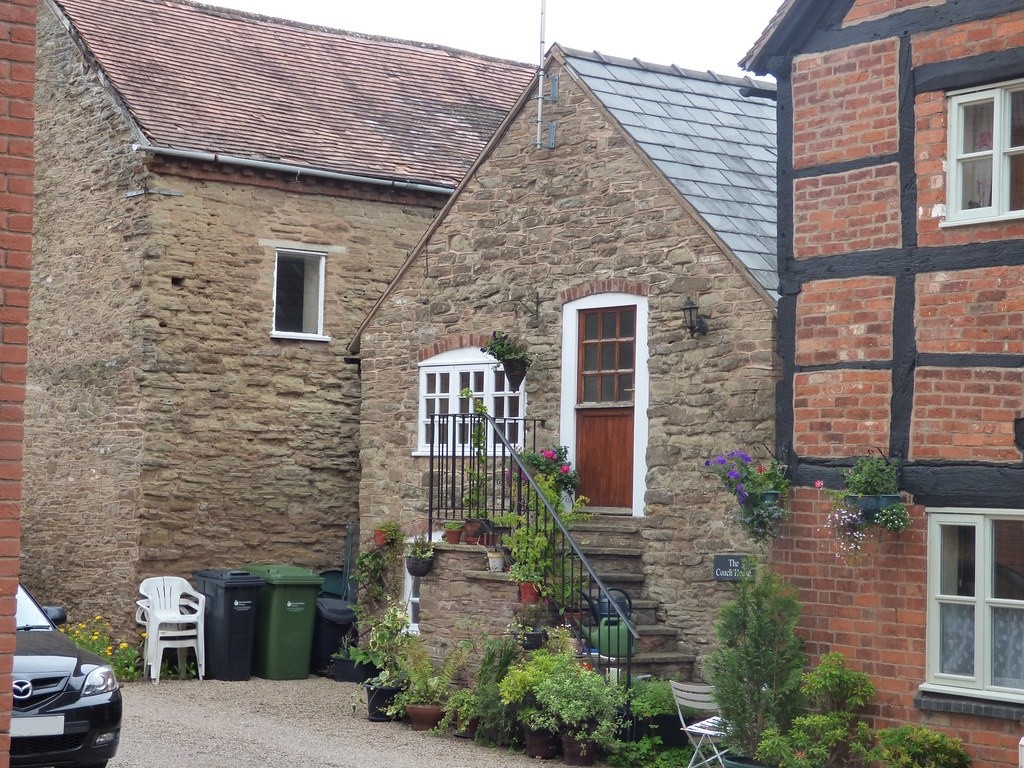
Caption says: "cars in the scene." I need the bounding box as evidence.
[7,576,123,768]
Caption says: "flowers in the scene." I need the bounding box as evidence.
[816,500,873,570]
[756,458,791,509]
[545,623,578,655]
[816,456,900,508]
[504,603,541,647]
[874,504,914,542]
[705,451,754,508]
[724,500,788,559]
[368,595,411,688]
[58,616,149,683]
[480,331,534,373]
[505,463,531,487]
[517,444,580,506]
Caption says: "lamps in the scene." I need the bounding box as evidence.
[680,296,708,339]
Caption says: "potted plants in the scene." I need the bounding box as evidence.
[533,663,634,767]
[443,522,463,544]
[487,512,528,546]
[331,646,380,685]
[424,688,484,739]
[501,526,548,605]
[462,468,492,545]
[495,648,567,760]
[376,634,473,731]
[541,569,590,630]
[373,519,398,546]
[403,529,435,577]
[701,546,810,768]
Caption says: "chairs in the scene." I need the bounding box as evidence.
[139,576,206,666]
[136,607,203,685]
[669,680,750,768]
[136,598,205,679]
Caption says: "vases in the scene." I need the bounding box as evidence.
[502,359,529,390]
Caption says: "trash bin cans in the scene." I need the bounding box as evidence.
[314,598,358,677]
[191,569,266,682]
[241,564,326,680]
